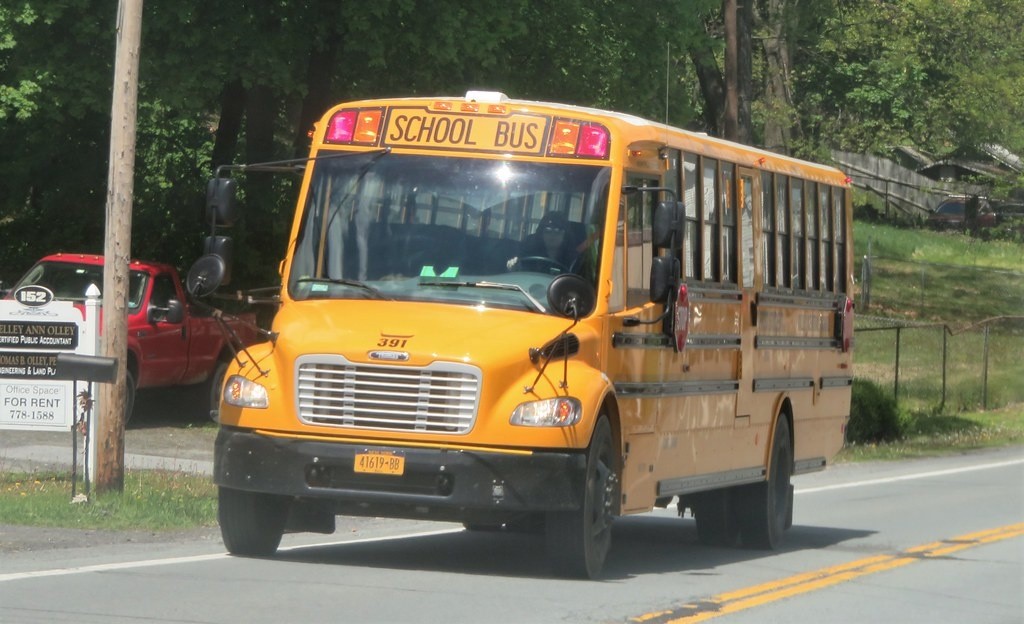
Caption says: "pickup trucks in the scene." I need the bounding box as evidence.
[1,252,262,424]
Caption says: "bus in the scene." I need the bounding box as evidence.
[181,90,855,576]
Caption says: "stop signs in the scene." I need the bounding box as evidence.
[675,286,690,350]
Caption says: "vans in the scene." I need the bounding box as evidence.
[918,195,1024,242]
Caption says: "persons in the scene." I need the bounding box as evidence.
[505,208,594,284]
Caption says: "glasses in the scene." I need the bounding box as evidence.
[541,226,565,234]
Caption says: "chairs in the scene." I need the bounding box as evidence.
[342,211,597,279]
